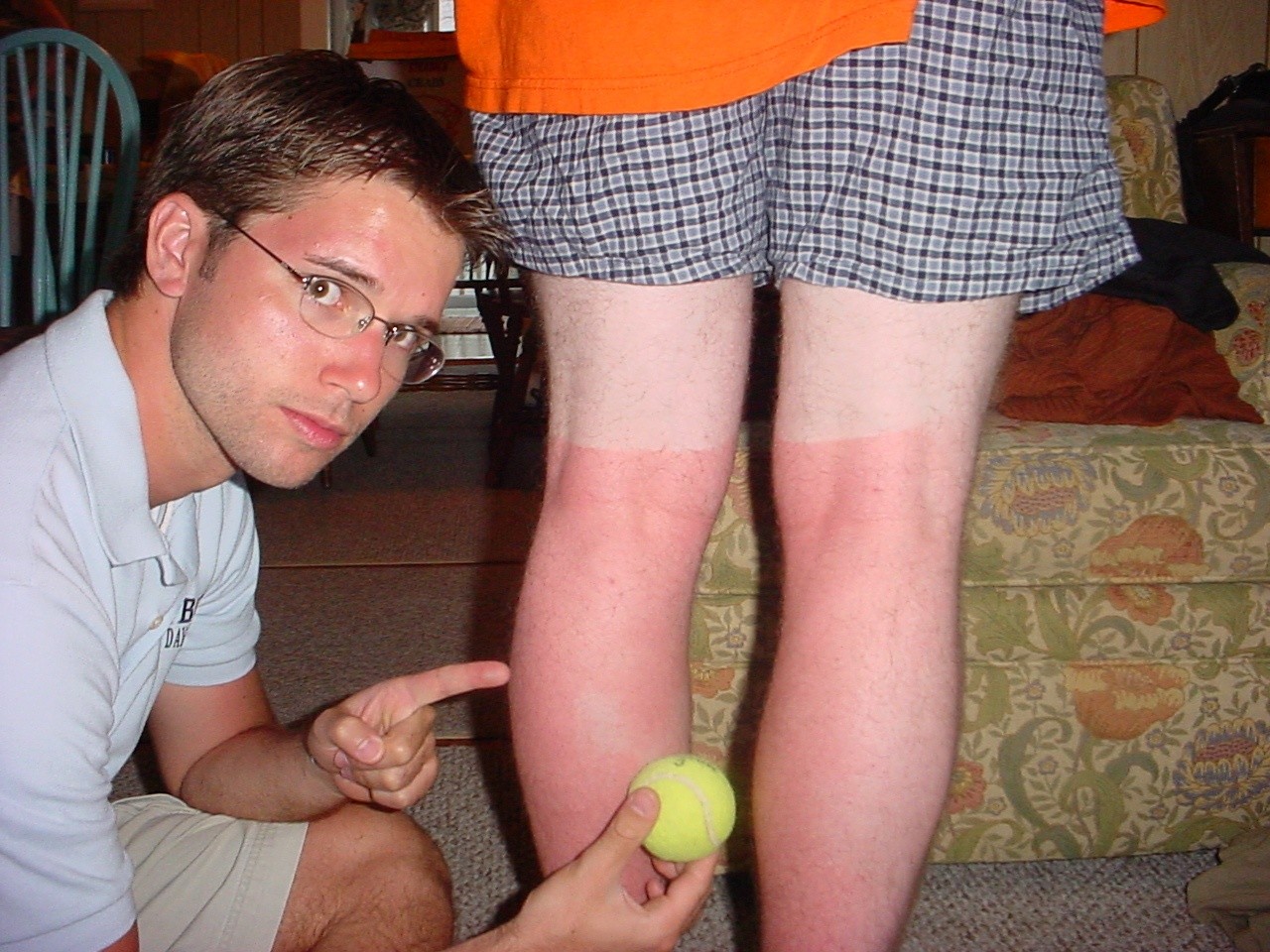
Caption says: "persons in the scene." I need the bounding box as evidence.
[1,51,718,952]
[452,2,1172,952]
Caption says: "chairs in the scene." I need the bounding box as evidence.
[0,28,140,326]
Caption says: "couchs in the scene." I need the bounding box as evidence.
[690,74,1270,877]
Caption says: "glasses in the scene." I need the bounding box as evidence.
[195,200,446,388]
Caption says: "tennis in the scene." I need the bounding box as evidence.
[626,754,738,861]
